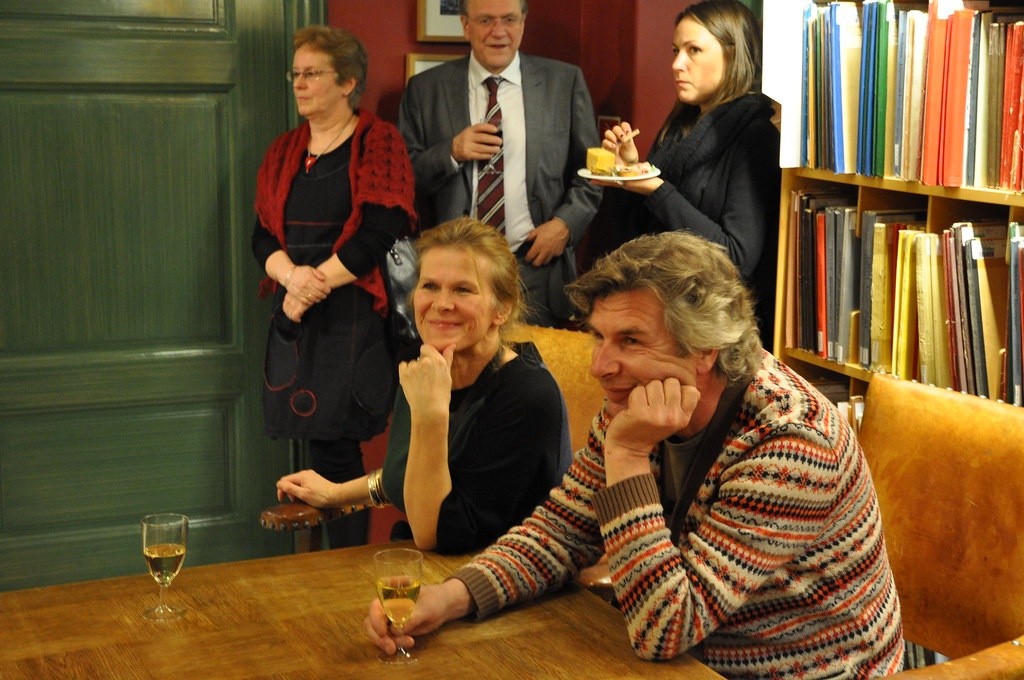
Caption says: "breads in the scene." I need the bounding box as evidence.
[587,147,615,171]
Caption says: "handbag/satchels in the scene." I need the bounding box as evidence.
[357,124,425,339]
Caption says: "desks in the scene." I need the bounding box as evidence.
[0,532,728,680]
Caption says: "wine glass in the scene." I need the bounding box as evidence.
[370,548,423,664]
[138,513,188,621]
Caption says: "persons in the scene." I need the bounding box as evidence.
[276,215,571,557]
[251,22,419,551]
[602,0,783,354]
[397,0,604,328]
[362,227,905,680]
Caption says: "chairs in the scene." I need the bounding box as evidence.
[492,320,658,596]
[850,369,1024,680]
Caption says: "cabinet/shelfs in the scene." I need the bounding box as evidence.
[771,0,1021,410]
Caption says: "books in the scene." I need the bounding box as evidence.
[916,220,1024,408]
[786,190,925,380]
[802,0,1024,196]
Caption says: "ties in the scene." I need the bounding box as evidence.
[478,77,506,237]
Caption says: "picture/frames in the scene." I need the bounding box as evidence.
[417,0,469,41]
[403,54,465,88]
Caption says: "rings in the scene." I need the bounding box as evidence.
[306,293,312,298]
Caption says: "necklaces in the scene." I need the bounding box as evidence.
[305,113,354,172]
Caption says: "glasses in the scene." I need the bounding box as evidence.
[285,65,341,84]
[464,11,526,27]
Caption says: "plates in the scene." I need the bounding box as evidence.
[578,165,661,180]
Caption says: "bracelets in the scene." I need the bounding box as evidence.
[367,468,392,510]
[284,265,297,289]
